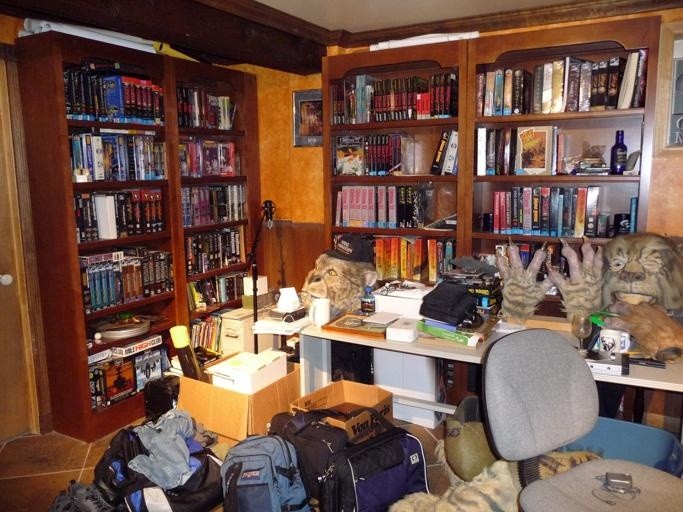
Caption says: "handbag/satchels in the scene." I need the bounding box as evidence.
[420,282,483,328]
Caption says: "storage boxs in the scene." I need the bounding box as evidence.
[175,350,393,449]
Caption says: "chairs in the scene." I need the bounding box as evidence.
[480,328,683,512]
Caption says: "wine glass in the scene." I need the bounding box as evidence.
[571,314,593,352]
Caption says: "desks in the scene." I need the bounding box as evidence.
[299,305,682,400]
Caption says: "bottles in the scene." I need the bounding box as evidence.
[610,129,628,176]
[360,287,376,317]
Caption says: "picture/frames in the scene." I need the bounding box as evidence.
[292,89,324,147]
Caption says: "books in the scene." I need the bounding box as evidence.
[179,183,244,227]
[72,188,165,243]
[69,126,166,181]
[417,270,503,349]
[86,334,171,409]
[176,86,237,130]
[474,49,646,177]
[336,186,455,283]
[63,61,165,125]
[76,247,175,314]
[178,135,240,179]
[164,345,222,379]
[183,224,243,277]
[493,185,637,281]
[190,270,247,350]
[331,69,458,177]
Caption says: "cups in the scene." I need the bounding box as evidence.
[599,329,631,354]
[308,298,331,327]
